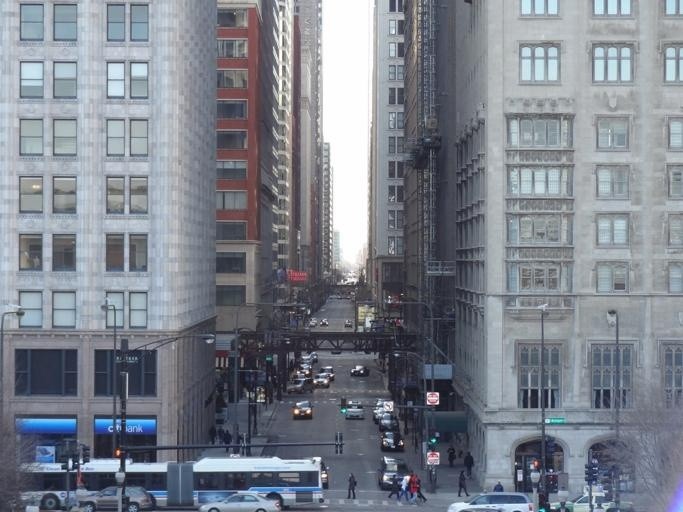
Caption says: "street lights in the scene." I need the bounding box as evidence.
[0,305,25,413]
[100,303,117,458]
[606,309,621,510]
[232,302,306,453]
[391,349,428,445]
[537,302,551,507]
[530,469,540,512]
[362,299,438,494]
[115,468,126,512]
[120,332,215,512]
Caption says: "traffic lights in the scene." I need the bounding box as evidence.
[83,446,90,462]
[601,471,612,500]
[548,474,558,492]
[591,462,597,480]
[340,399,346,413]
[60,454,67,470]
[72,451,77,469]
[534,460,539,467]
[538,494,545,506]
[114,447,126,455]
[584,463,591,482]
[430,439,436,449]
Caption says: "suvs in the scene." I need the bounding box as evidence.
[77,484,155,512]
[447,491,534,512]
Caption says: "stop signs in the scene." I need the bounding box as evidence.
[426,452,439,465]
[427,392,439,405]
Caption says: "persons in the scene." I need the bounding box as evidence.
[208,425,242,453]
[493,481,503,492]
[465,452,473,479]
[447,445,455,467]
[458,471,470,497]
[388,473,427,507]
[346,473,355,499]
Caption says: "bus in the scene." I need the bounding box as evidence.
[16,457,323,510]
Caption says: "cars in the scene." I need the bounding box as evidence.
[349,364,369,378]
[538,492,633,512]
[343,399,364,420]
[287,352,333,419]
[370,397,403,451]
[307,316,328,328]
[321,462,331,490]
[200,490,281,512]
[344,319,352,328]
[376,455,411,490]
